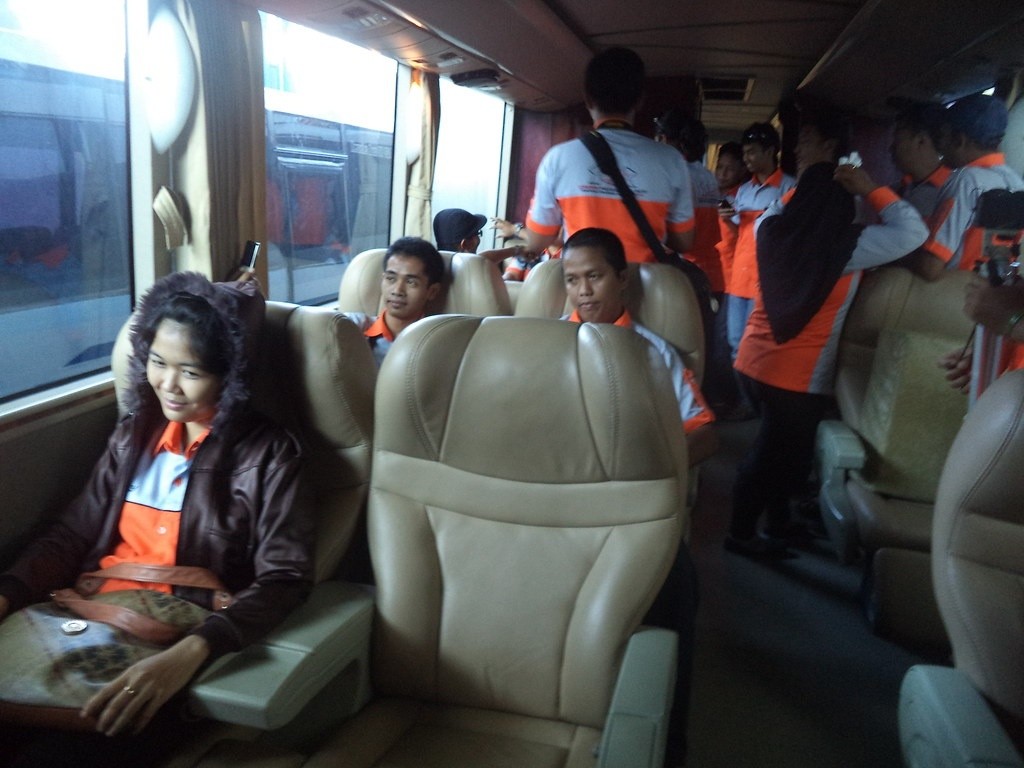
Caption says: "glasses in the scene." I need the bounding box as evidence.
[746,130,770,141]
[463,228,483,245]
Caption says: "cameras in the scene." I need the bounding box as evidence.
[974,188,1024,230]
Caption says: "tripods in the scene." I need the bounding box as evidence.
[965,230,1021,412]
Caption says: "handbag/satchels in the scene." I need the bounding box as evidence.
[660,249,718,376]
[0,564,235,726]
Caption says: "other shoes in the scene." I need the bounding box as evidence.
[726,403,758,422]
[722,531,792,562]
[764,518,809,542]
[808,518,828,537]
[800,498,822,519]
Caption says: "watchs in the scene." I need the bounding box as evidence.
[514,223,523,238]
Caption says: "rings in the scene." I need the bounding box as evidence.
[851,165,857,170]
[124,687,138,697]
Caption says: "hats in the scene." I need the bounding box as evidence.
[947,93,1008,149]
[433,208,487,247]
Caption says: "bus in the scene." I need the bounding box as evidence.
[0,59,392,401]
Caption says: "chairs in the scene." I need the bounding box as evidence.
[113,299,379,768]
[503,279,524,313]
[504,240,529,250]
[514,258,708,405]
[820,265,996,648]
[897,365,1024,768]
[180,311,688,768]
[337,248,515,320]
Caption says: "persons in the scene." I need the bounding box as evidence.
[491,42,1024,768]
[230,239,443,366]
[434,208,540,282]
[0,273,326,768]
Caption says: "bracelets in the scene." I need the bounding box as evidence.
[1006,309,1024,337]
[514,245,520,258]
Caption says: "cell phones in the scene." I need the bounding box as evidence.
[235,240,260,275]
[719,200,736,218]
